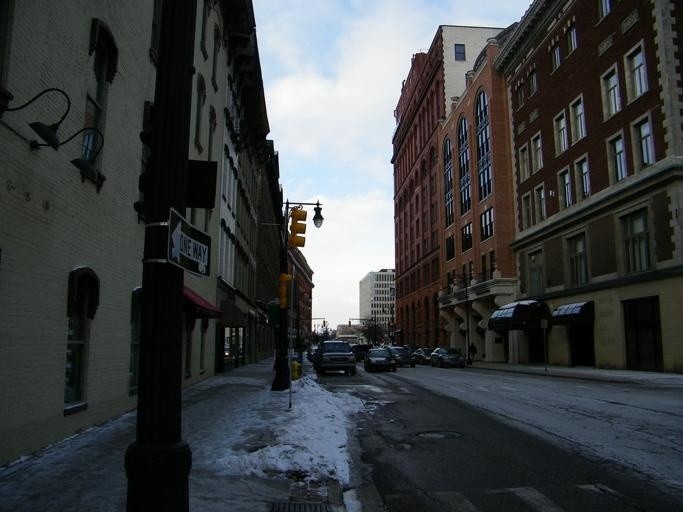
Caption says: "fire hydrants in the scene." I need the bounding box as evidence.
[290,358,302,381]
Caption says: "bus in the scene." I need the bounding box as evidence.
[333,334,361,349]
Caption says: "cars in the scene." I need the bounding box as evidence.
[306,340,466,376]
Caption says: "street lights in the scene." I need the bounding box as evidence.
[382,306,394,345]
[271,198,325,392]
[449,272,473,368]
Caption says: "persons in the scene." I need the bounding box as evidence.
[470,342,477,363]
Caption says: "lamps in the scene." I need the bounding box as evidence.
[2,87,106,185]
[283,199,324,229]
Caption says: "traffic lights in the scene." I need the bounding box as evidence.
[289,208,307,248]
[279,272,294,309]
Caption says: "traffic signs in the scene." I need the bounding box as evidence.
[165,206,212,279]
